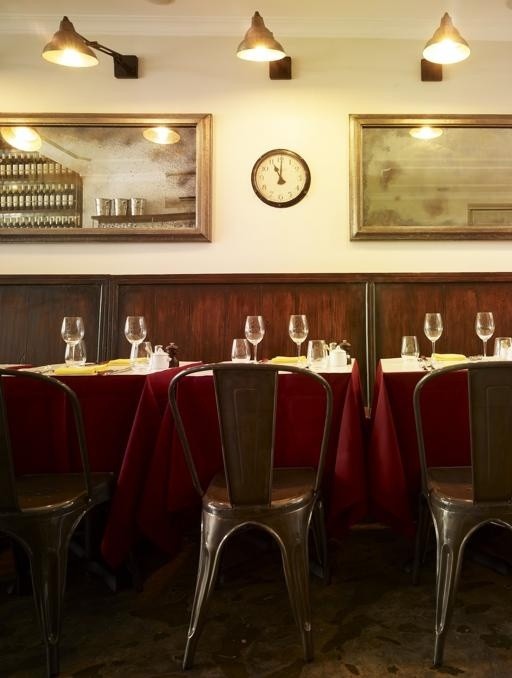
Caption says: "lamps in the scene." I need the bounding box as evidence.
[42,15,138,80]
[421,11,470,83]
[237,10,292,80]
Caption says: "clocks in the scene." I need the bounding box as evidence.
[250,149,311,208]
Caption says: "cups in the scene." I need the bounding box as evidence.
[124,315,154,375]
[492,337,512,362]
[94,197,112,218]
[474,311,496,362]
[308,339,330,370]
[231,338,250,362]
[130,197,146,216]
[114,197,129,216]
[400,335,420,361]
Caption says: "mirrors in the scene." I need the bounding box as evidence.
[346,114,512,241]
[0,113,213,243]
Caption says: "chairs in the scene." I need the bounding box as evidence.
[411,360,512,669]
[1,371,115,676]
[167,363,332,670]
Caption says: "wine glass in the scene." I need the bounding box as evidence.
[60,316,88,368]
[289,314,310,368]
[423,312,445,364]
[245,314,267,366]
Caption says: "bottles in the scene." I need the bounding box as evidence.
[0,151,76,229]
[150,342,179,372]
[329,339,353,369]
